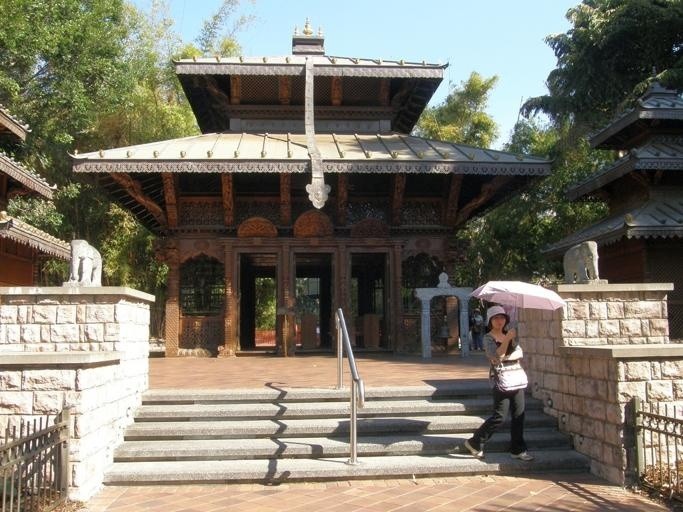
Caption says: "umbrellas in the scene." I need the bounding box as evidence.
[468,280,568,347]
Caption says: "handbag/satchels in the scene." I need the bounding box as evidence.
[494,363,529,393]
[472,325,481,334]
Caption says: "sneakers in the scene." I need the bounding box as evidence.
[464,440,485,460]
[509,449,535,461]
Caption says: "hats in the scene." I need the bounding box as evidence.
[474,308,481,315]
[486,306,510,330]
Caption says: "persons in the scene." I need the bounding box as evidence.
[463,305,534,461]
[466,307,491,351]
[431,322,450,352]
